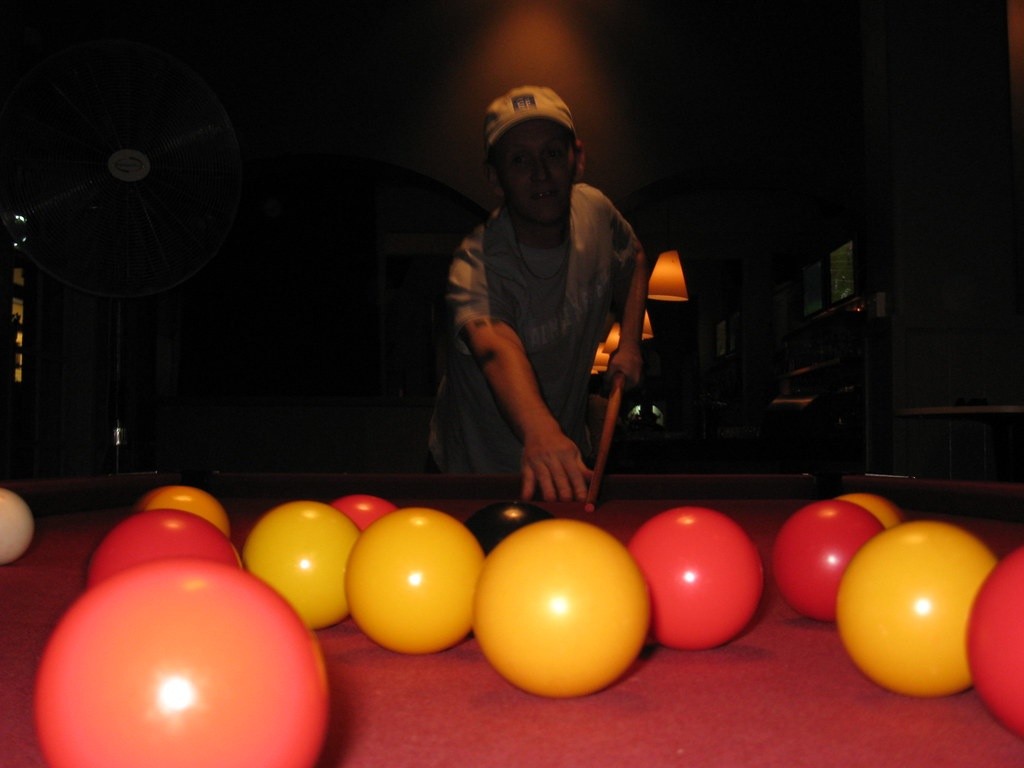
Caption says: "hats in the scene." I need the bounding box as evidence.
[483,86,577,161]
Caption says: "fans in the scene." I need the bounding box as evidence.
[0,38,244,473]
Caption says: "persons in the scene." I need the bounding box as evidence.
[423,84,649,503]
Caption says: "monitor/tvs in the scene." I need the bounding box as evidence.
[799,239,857,321]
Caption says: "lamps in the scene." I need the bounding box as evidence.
[646,249,689,303]
[641,308,655,341]
[590,321,621,376]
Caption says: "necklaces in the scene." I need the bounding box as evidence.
[513,223,571,279]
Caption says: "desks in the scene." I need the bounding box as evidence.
[0,472,1024,768]
[894,405,1024,482]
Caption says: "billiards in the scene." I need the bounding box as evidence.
[31,481,331,768]
[1,486,36,568]
[238,489,767,703]
[772,489,1024,743]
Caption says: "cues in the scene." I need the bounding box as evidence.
[583,372,629,514]
